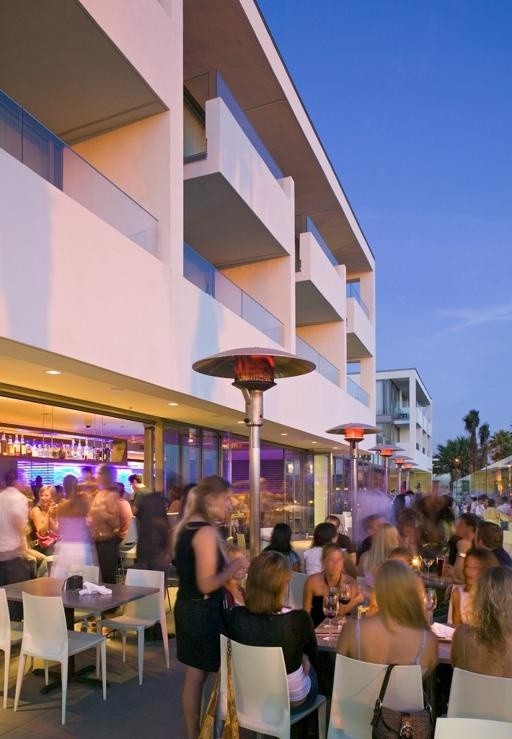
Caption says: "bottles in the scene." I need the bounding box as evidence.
[0,430,110,462]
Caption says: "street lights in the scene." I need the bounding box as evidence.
[370,441,420,500]
[455,458,461,503]
[191,343,316,561]
[324,422,382,542]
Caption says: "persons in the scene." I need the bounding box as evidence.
[449,564,511,676]
[266,490,511,626]
[340,560,438,685]
[1,461,172,587]
[226,549,317,707]
[172,474,248,739]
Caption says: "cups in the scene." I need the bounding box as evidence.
[437,555,445,577]
[228,543,246,580]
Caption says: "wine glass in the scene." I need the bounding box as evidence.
[426,588,438,624]
[423,558,436,586]
[323,596,340,644]
[340,584,351,620]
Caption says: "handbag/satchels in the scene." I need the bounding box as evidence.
[198,704,239,739]
[371,699,433,739]
